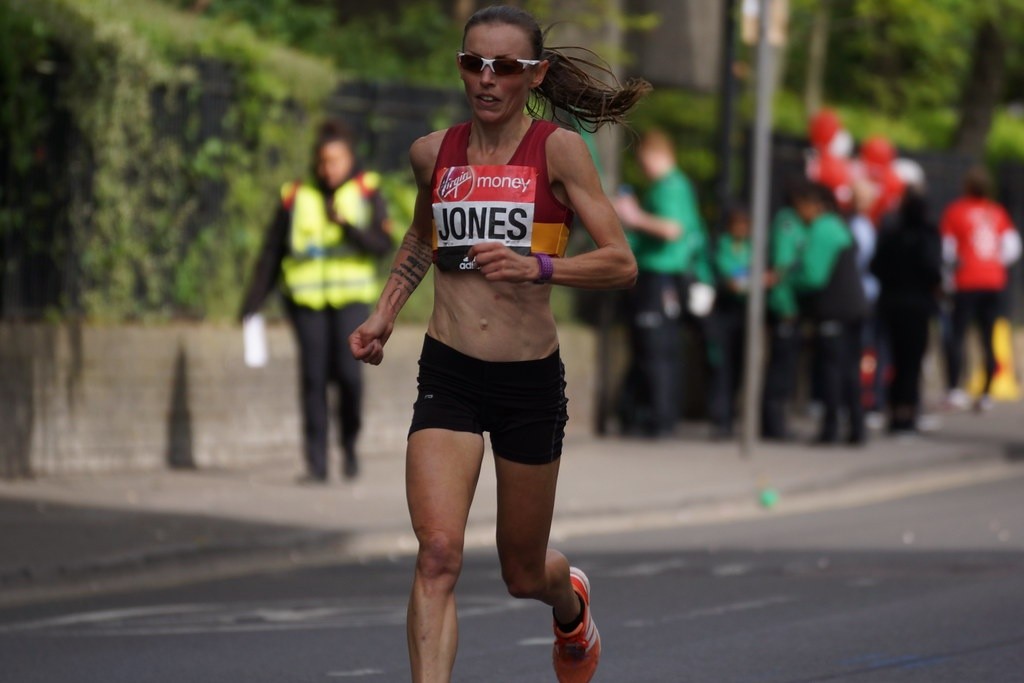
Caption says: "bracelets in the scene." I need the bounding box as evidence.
[533,254,552,285]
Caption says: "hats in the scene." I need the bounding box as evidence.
[891,160,922,187]
[860,135,893,166]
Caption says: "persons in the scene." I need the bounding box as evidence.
[349,6,638,683]
[617,128,1022,445]
[238,120,391,480]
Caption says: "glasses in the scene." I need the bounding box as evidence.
[455,52,542,77]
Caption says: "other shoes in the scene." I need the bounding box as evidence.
[813,417,836,442]
[310,465,327,481]
[847,413,866,445]
[343,453,355,477]
[886,406,920,432]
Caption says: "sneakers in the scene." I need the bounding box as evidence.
[553,566,600,683]
